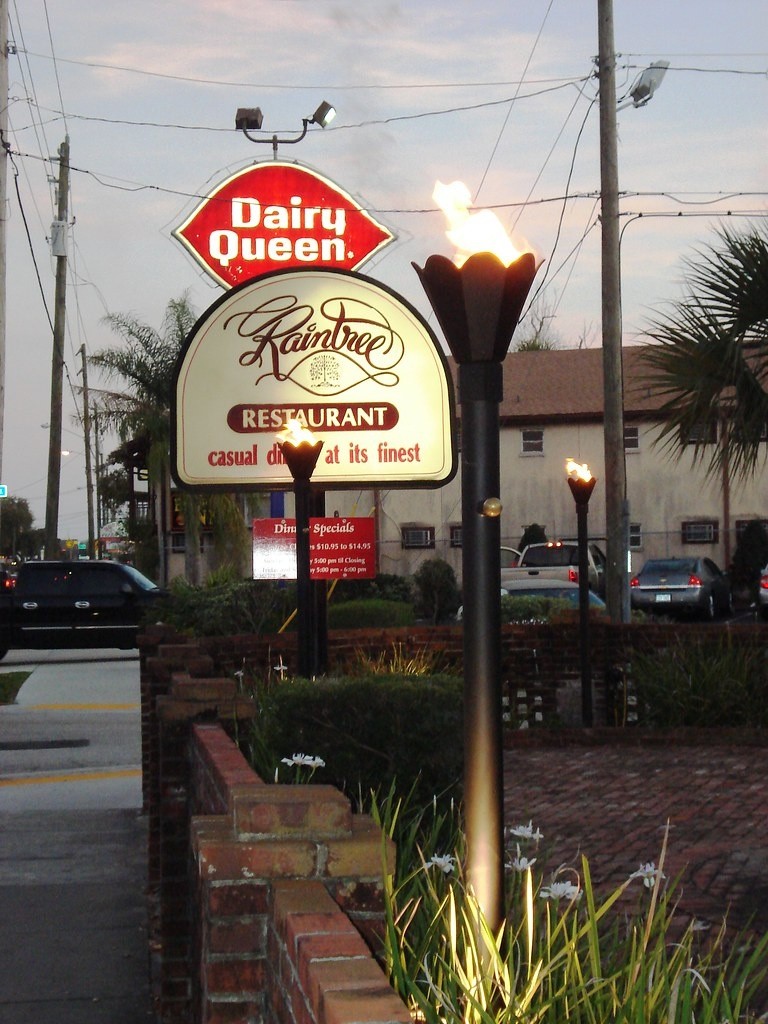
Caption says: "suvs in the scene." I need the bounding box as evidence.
[13,559,161,630]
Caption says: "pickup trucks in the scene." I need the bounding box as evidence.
[498,539,608,598]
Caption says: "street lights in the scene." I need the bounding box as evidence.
[40,422,106,559]
[234,96,336,163]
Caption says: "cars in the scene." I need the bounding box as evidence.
[499,578,609,612]
[628,554,731,622]
[0,550,32,616]
